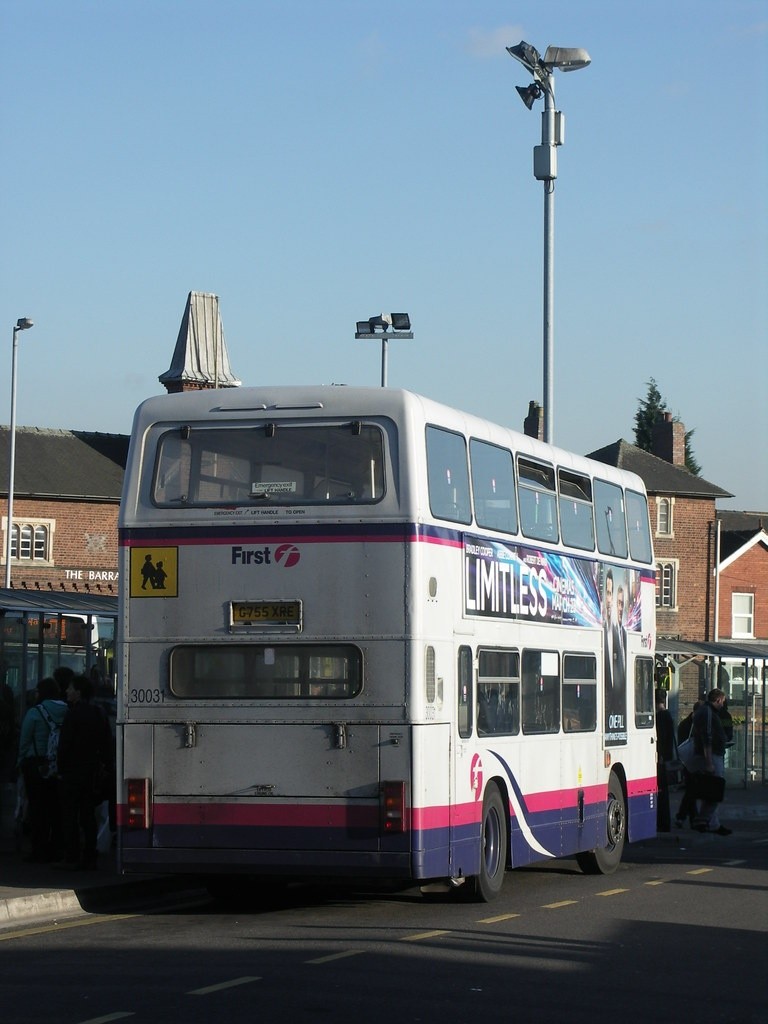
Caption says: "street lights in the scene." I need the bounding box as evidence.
[355,312,415,392]
[505,40,593,440]
[8,317,35,591]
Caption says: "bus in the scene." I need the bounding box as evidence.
[2,611,101,692]
[108,387,671,905]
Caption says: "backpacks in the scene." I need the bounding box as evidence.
[35,703,70,790]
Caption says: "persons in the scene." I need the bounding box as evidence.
[656,689,675,834]
[15,677,111,869]
[677,689,734,836]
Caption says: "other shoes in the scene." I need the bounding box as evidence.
[675,820,685,829]
[704,824,732,836]
[21,849,48,864]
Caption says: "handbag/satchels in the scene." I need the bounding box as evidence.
[686,771,726,805]
[677,737,695,773]
